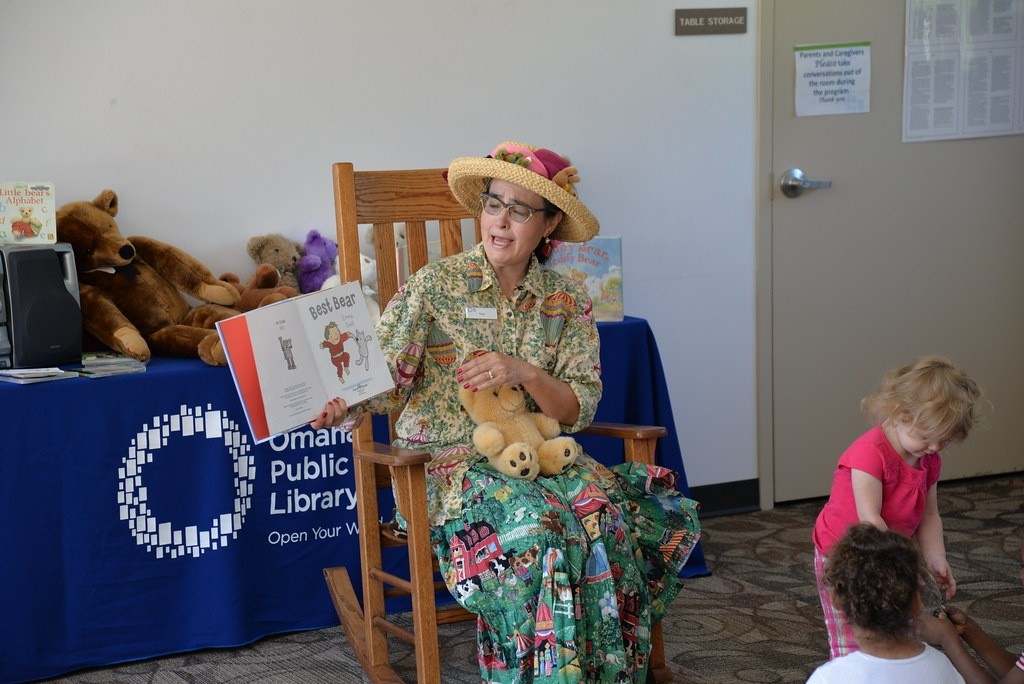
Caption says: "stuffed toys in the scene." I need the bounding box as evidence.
[57,188,408,366]
[459,381,577,479]
[10,206,41,238]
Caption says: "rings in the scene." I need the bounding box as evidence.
[488,370,493,379]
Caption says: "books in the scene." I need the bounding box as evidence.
[214,279,395,446]
[543,235,624,320]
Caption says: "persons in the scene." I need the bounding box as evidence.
[805,524,965,684]
[913,603,1024,684]
[814,356,981,662]
[311,142,702,684]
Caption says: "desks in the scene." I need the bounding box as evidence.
[0,315,711,684]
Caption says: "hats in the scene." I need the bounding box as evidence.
[447,142,600,243]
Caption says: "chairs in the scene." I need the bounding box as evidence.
[323,160,677,684]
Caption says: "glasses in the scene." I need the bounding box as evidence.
[480,192,548,224]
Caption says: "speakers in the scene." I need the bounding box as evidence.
[0,240,83,366]
